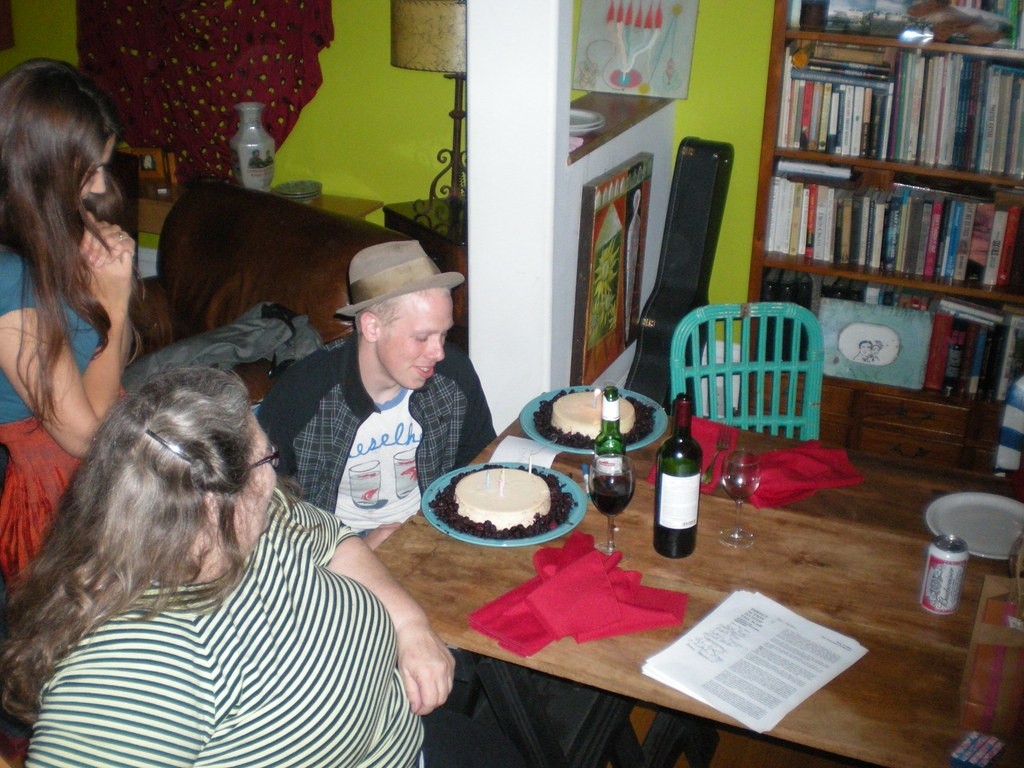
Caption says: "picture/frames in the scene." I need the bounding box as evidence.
[133,148,163,176]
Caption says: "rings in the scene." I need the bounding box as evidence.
[118,232,123,240]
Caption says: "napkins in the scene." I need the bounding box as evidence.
[738,442,863,507]
[648,416,740,495]
[472,533,686,657]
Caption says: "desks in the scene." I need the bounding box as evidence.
[138,195,385,236]
[384,200,470,330]
[370,420,1024,768]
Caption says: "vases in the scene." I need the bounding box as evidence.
[230,100,275,192]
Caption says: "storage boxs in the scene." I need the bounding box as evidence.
[964,575,1024,735]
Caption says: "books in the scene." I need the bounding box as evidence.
[777,40,1024,175]
[761,267,1024,400]
[765,159,1024,286]
[949,0,1024,52]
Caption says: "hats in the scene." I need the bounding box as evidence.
[334,240,466,316]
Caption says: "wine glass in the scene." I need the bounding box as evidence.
[588,454,637,567]
[718,448,762,550]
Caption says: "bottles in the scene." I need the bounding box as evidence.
[228,101,276,193]
[653,393,703,559]
[593,387,627,458]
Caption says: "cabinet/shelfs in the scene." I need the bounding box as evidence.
[745,1,1024,487]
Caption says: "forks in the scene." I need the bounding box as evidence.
[701,421,733,484]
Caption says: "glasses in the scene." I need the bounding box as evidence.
[241,445,280,475]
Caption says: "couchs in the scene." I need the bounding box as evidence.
[131,173,414,403]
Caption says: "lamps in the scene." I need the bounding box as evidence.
[388,0,469,261]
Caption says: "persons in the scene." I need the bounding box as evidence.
[0,57,135,768]
[255,240,497,552]
[0,366,524,768]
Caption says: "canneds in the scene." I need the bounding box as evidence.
[921,535,969,615]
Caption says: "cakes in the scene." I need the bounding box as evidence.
[429,463,575,539]
[533,388,654,450]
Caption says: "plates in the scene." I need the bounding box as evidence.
[568,108,606,136]
[421,461,587,548]
[274,180,323,200]
[924,491,1024,560]
[519,382,668,455]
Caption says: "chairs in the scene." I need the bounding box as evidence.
[670,302,824,442]
